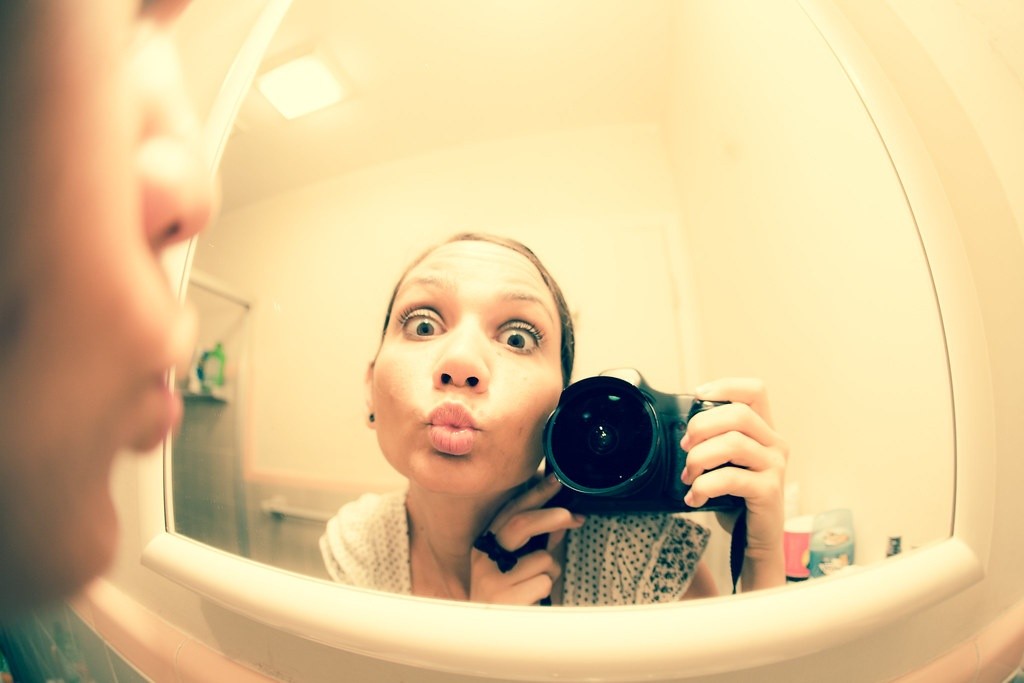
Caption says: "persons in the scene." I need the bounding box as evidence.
[0,0,211,597]
[321,232,790,606]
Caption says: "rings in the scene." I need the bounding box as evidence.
[473,530,517,573]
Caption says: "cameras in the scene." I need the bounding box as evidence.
[540,368,744,517]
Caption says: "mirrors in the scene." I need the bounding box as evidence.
[134,0,998,683]
